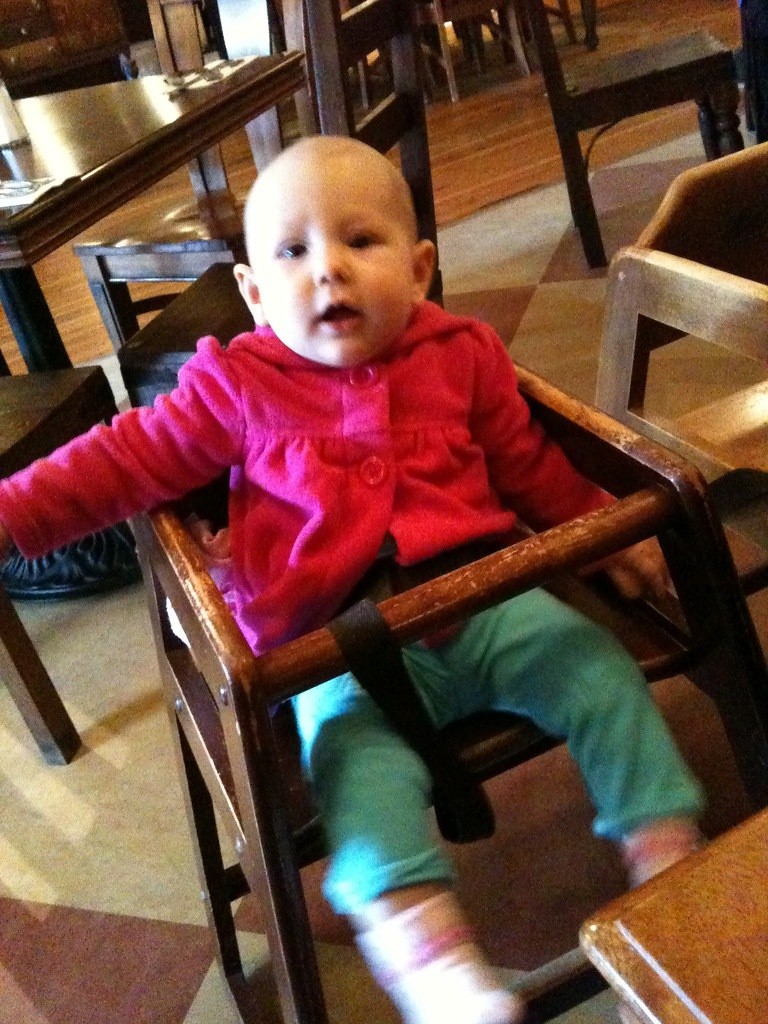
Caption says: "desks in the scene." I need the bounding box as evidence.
[0,46,304,394]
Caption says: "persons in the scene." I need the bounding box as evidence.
[0,134,710,1024]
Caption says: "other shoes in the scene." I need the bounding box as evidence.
[354,891,523,1024]
[624,823,704,889]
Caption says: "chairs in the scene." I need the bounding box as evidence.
[2,1,767,1023]
